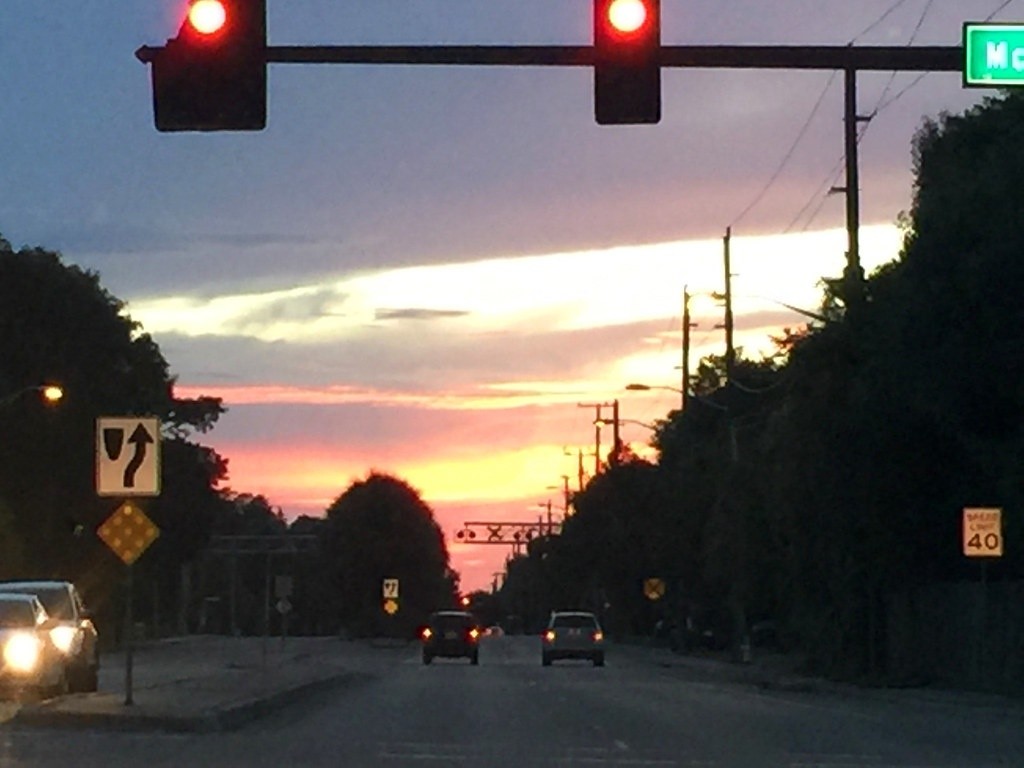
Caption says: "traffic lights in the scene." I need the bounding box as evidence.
[593,0,663,126]
[135,0,268,135]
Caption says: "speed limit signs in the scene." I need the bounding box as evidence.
[963,509,1004,557]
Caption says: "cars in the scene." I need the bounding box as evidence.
[0,581,100,693]
[540,611,605,668]
[0,593,69,700]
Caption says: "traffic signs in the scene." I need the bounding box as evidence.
[961,22,1024,88]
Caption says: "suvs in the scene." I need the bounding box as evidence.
[421,609,479,666]
